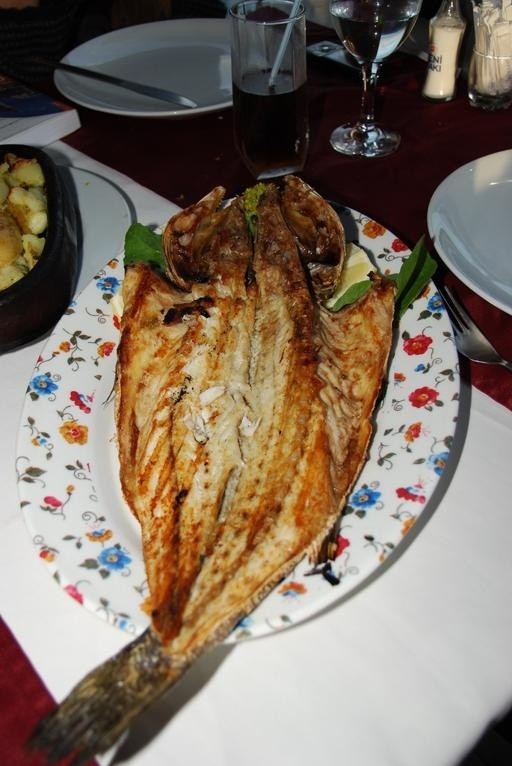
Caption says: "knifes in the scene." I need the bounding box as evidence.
[45,56,198,107]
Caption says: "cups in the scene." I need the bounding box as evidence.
[464,47,511,111]
[230,1,311,178]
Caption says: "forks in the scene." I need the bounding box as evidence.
[436,284,510,373]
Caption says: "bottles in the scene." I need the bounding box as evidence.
[421,0,468,102]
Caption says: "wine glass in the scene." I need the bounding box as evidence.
[327,0,422,157]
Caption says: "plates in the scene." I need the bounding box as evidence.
[20,197,463,642]
[54,19,289,117]
[4,167,134,377]
[427,147,511,319]
